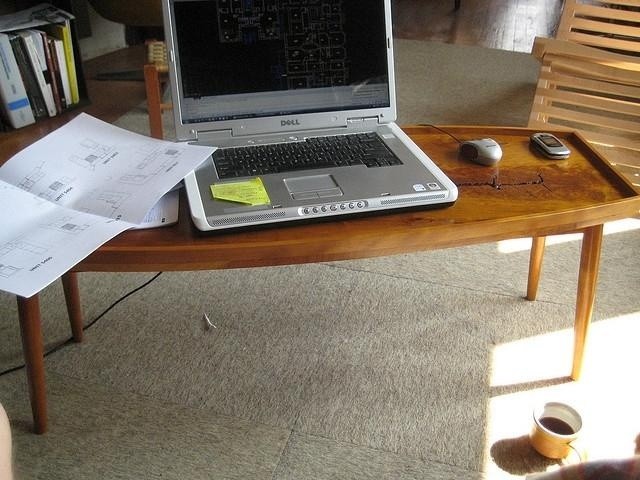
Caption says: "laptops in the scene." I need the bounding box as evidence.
[160,0,459,234]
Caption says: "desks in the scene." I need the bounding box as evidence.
[0,44,640,434]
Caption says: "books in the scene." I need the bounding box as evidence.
[0,4,80,130]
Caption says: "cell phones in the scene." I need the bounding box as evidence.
[528,132,572,160]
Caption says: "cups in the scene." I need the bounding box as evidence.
[529,401,589,466]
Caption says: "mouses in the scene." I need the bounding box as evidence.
[462,136,502,166]
